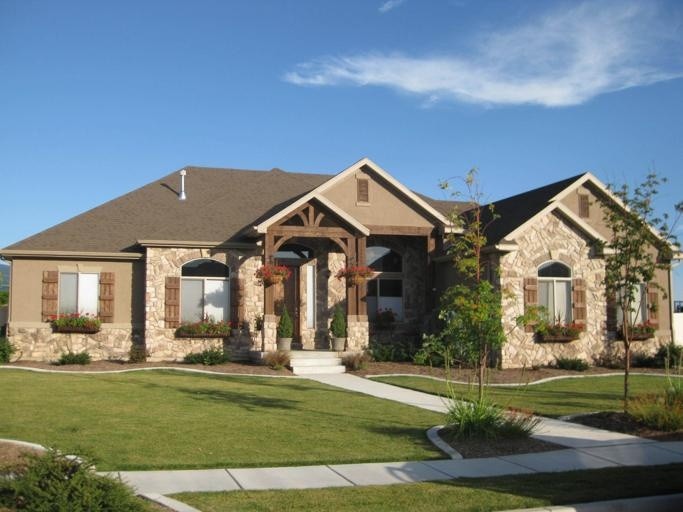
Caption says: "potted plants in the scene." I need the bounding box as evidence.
[329,302,346,351]
[277,304,293,350]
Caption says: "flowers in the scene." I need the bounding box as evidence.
[255,265,292,279]
[335,266,374,275]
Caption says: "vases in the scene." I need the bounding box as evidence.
[348,277,364,285]
[268,275,281,283]
